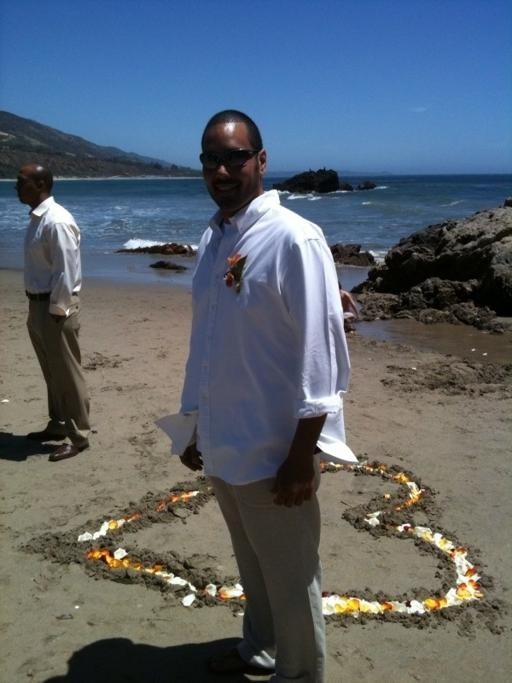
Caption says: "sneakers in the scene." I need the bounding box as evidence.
[207,647,276,675]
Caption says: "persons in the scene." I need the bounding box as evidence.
[13,162,94,460]
[151,107,356,683]
[337,280,360,335]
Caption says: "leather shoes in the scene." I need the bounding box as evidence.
[26,430,66,442]
[49,443,90,461]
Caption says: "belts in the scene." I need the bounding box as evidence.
[25,290,79,301]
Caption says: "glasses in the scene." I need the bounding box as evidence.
[200,149,259,170]
